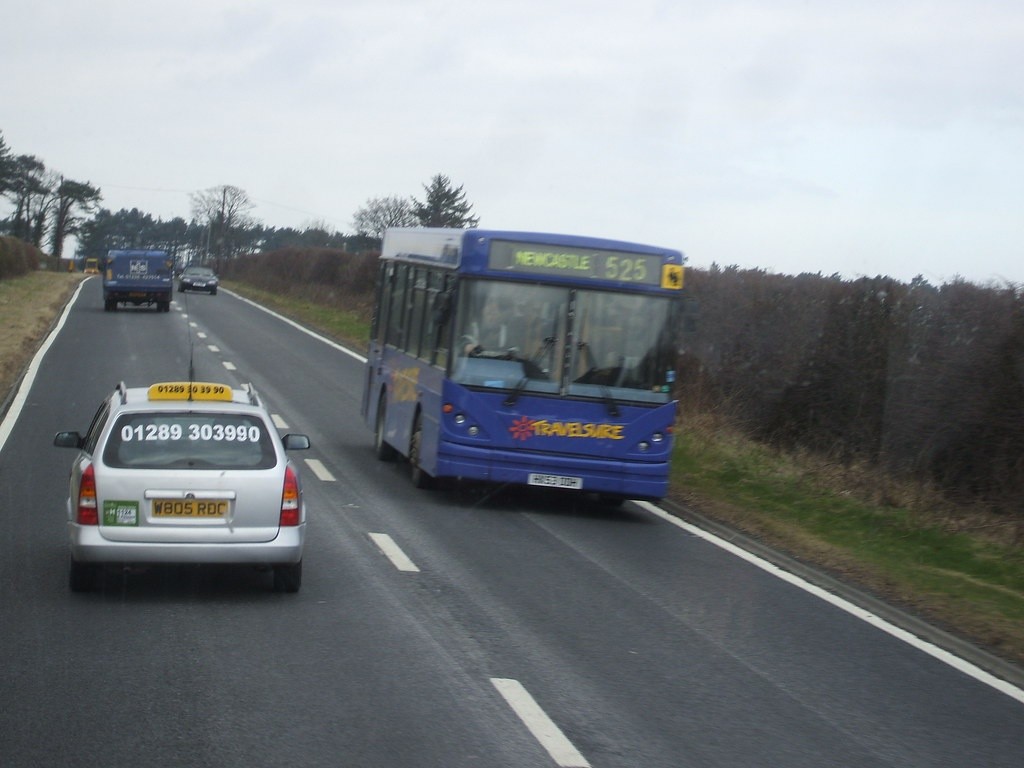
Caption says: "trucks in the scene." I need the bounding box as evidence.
[98,249,173,313]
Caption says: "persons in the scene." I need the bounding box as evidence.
[461,304,520,357]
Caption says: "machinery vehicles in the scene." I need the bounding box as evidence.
[84,257,99,275]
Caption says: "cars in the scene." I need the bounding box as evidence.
[178,266,220,295]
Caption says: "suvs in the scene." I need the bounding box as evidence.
[51,380,312,593]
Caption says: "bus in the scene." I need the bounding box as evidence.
[361,228,700,513]
